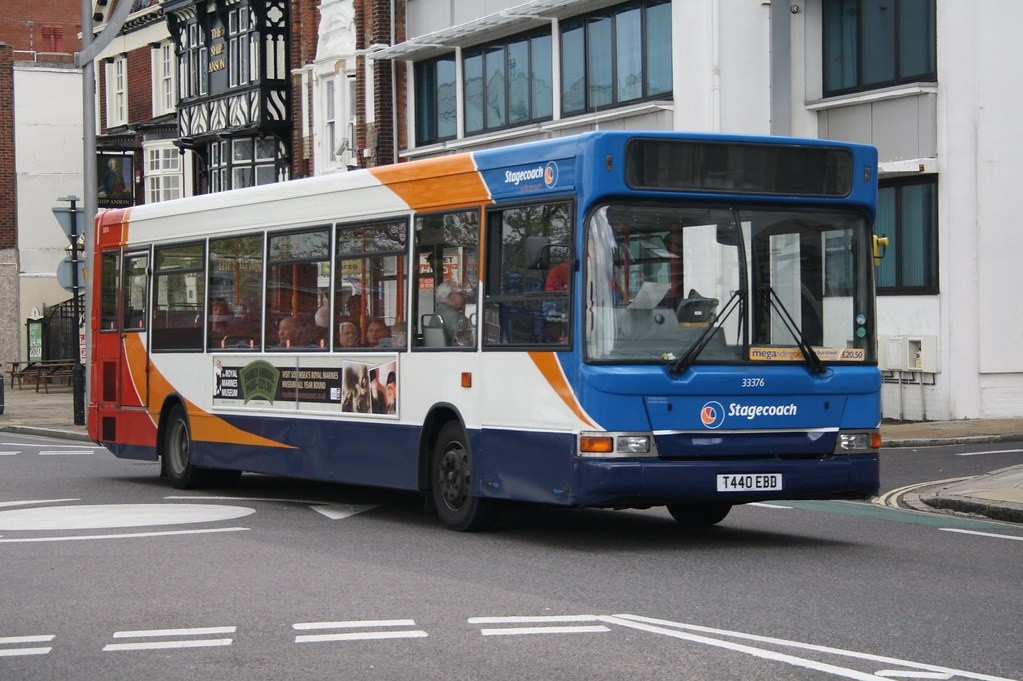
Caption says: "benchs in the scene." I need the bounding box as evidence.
[5,370,73,378]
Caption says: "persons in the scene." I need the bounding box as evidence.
[301,325,323,347]
[366,320,392,347]
[340,322,357,347]
[545,236,623,301]
[213,298,260,347]
[315,307,329,346]
[103,158,131,198]
[277,318,300,346]
[349,300,371,331]
[428,281,472,345]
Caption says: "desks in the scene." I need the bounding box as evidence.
[32,364,76,394]
[7,358,74,390]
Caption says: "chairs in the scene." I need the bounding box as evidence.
[221,335,256,348]
[421,314,450,348]
[166,303,205,327]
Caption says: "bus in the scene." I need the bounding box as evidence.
[87,129,890,531]
